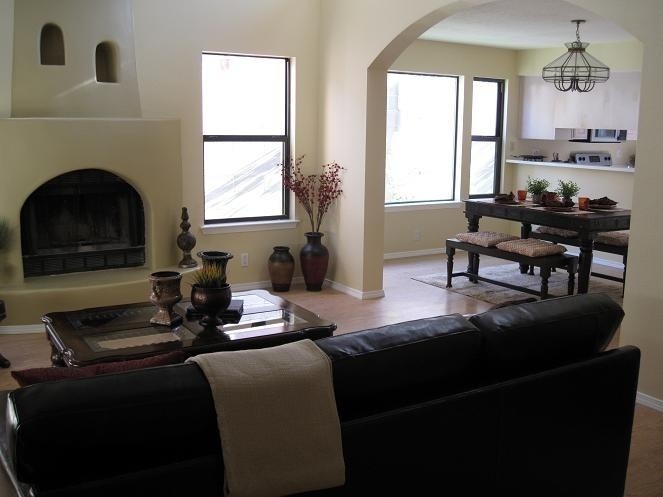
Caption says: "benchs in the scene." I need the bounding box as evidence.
[529,230,628,297]
[446,238,578,300]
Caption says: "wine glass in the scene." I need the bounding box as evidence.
[145,268,185,328]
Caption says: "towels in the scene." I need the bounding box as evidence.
[187,300,244,315]
[185,340,346,497]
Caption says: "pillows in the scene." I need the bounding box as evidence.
[11,349,183,387]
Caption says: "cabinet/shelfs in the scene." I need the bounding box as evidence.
[519,72,641,141]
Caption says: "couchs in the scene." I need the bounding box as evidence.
[0,294,642,497]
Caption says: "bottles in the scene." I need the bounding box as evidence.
[175,207,197,267]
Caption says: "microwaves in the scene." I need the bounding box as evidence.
[590,128,621,142]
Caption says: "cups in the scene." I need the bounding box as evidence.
[196,249,234,271]
[578,197,591,209]
[517,189,527,200]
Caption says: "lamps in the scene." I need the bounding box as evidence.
[542,20,609,92]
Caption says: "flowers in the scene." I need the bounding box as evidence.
[277,154,344,234]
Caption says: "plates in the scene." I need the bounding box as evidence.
[589,203,616,208]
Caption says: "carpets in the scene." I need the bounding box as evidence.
[411,262,624,305]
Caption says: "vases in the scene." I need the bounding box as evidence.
[300,232,329,291]
[197,251,233,282]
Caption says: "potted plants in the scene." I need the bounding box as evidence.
[185,262,232,311]
[526,176,550,203]
[554,180,581,207]
